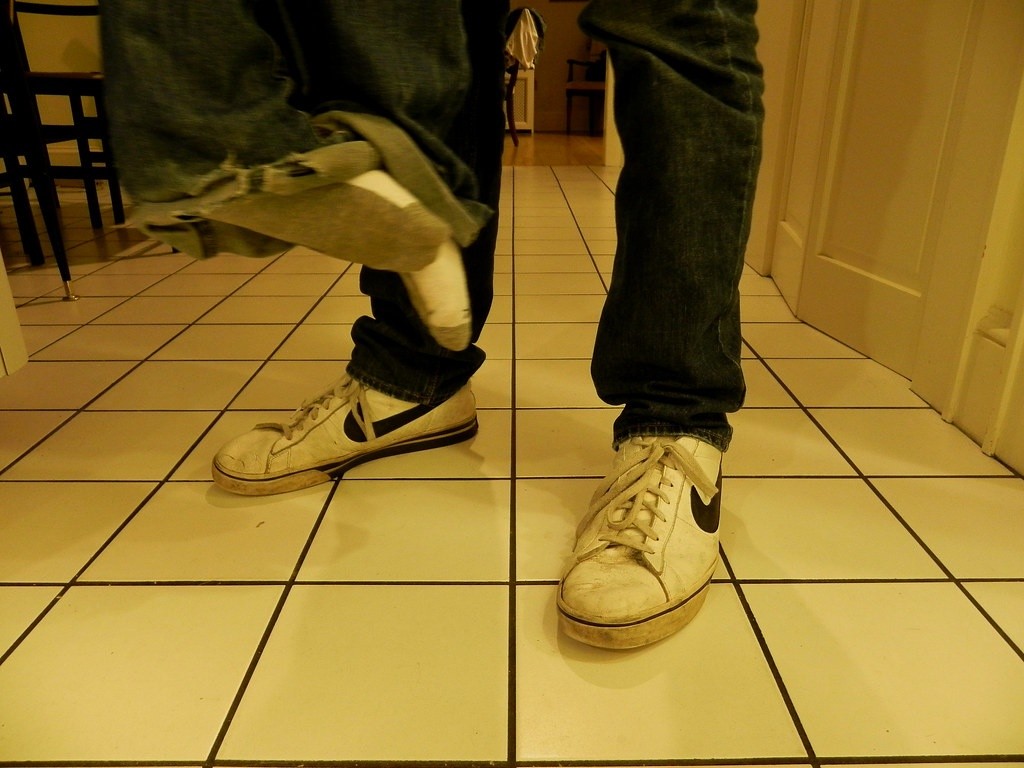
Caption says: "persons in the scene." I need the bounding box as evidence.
[210,0,765,650]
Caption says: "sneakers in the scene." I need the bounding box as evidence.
[213,372,484,494]
[556,433,724,649]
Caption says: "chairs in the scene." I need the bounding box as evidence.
[0,0,129,266]
[503,7,529,146]
[563,38,608,134]
